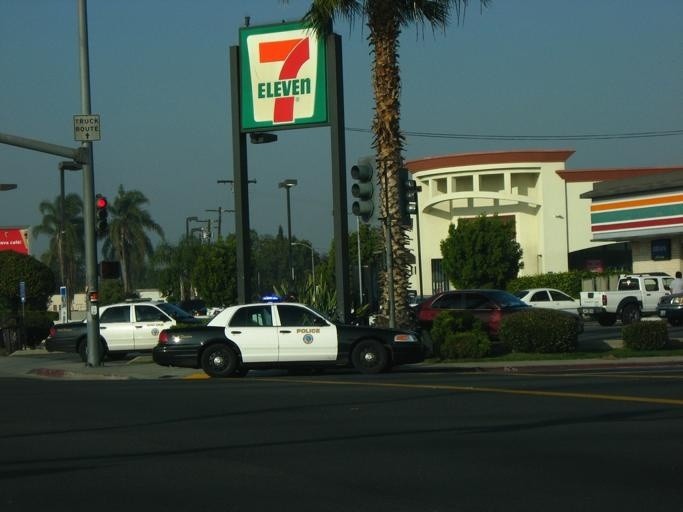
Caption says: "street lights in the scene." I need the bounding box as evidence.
[290,243,314,306]
[58,162,82,292]
[185,181,255,305]
[278,179,297,291]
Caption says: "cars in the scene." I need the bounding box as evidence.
[45,300,214,362]
[402,288,582,341]
[656,292,683,326]
[152,296,424,373]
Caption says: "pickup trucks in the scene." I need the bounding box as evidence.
[579,272,676,325]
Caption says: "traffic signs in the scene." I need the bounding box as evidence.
[72,114,99,141]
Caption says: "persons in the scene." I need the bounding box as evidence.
[667,271,682,295]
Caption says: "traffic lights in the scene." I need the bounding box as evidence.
[351,157,380,224]
[95,197,107,233]
[99,262,118,279]
